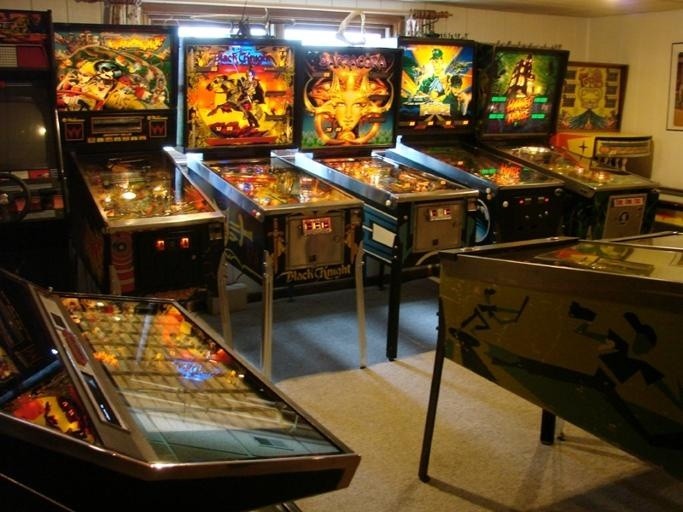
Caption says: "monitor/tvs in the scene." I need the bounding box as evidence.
[1,84,55,176]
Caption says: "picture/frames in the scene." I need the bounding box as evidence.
[666,42,683,131]
[555,61,631,135]
